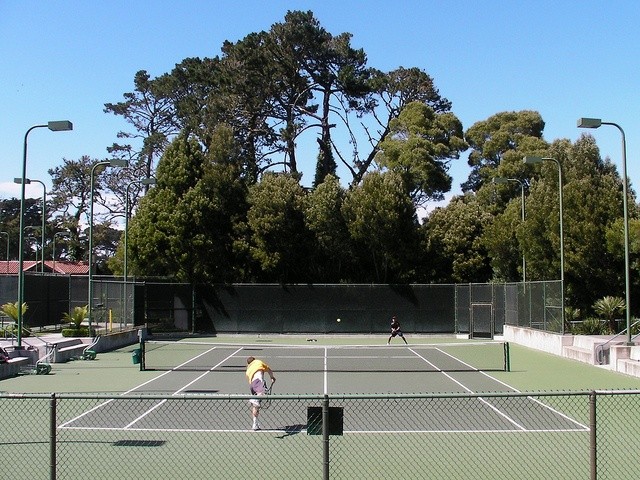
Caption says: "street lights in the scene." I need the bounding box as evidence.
[119,177,158,329]
[492,177,534,330]
[14,177,48,273]
[576,117,634,346]
[15,121,73,347]
[86,159,129,336]
[524,154,570,334]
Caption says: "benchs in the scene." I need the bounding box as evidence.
[0,347,39,379]
[51,337,89,363]
[561,334,608,364]
[608,344,640,375]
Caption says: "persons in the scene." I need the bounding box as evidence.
[387,316,408,345]
[245,357,276,430]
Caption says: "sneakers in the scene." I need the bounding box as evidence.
[252,425,261,431]
[249,399,260,408]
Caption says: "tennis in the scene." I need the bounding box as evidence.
[336,318,340,322]
